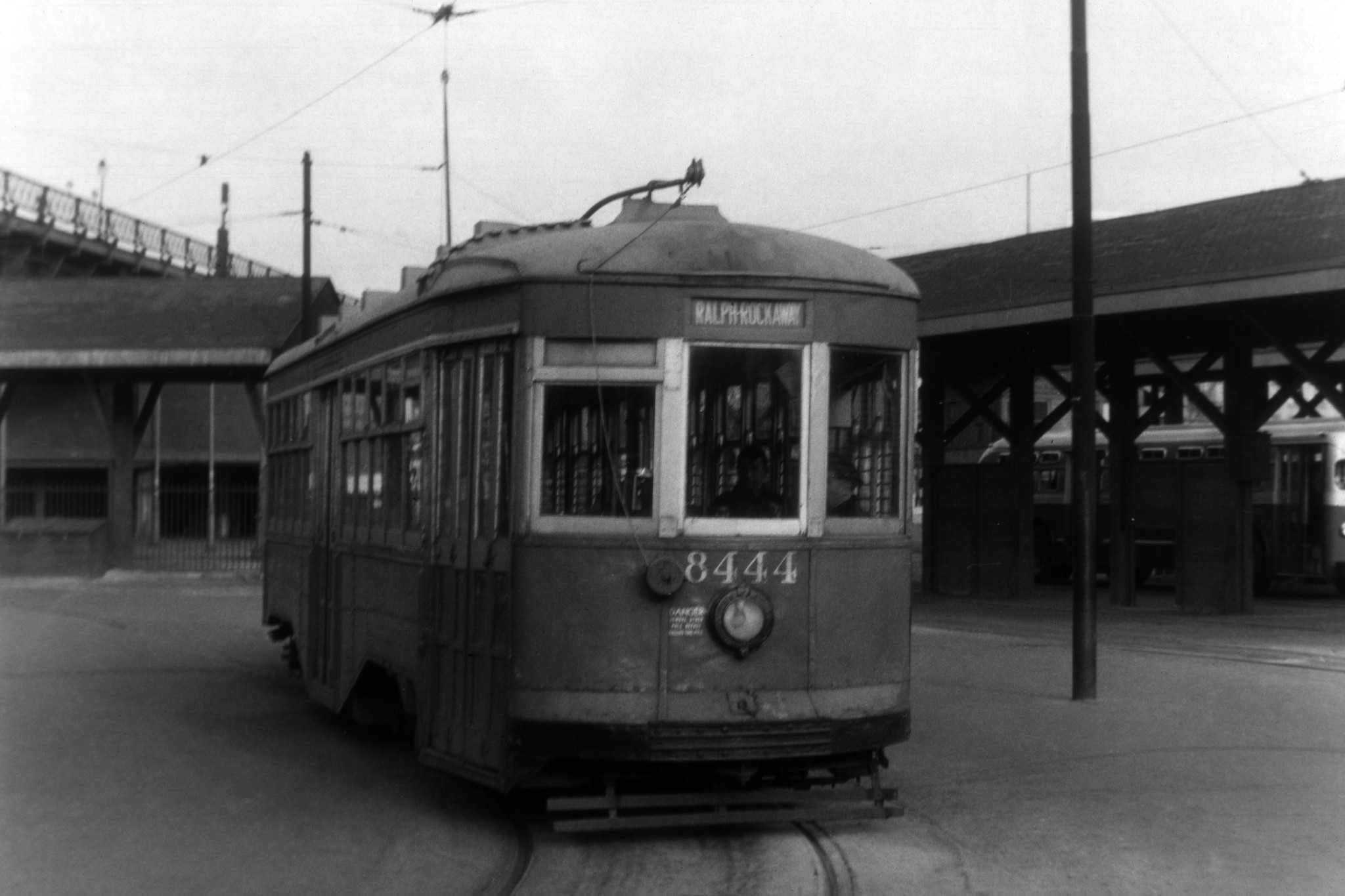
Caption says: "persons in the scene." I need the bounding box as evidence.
[827,453,863,517]
[713,446,783,517]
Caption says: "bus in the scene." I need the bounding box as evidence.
[261,159,924,839]
[980,421,1345,597]
